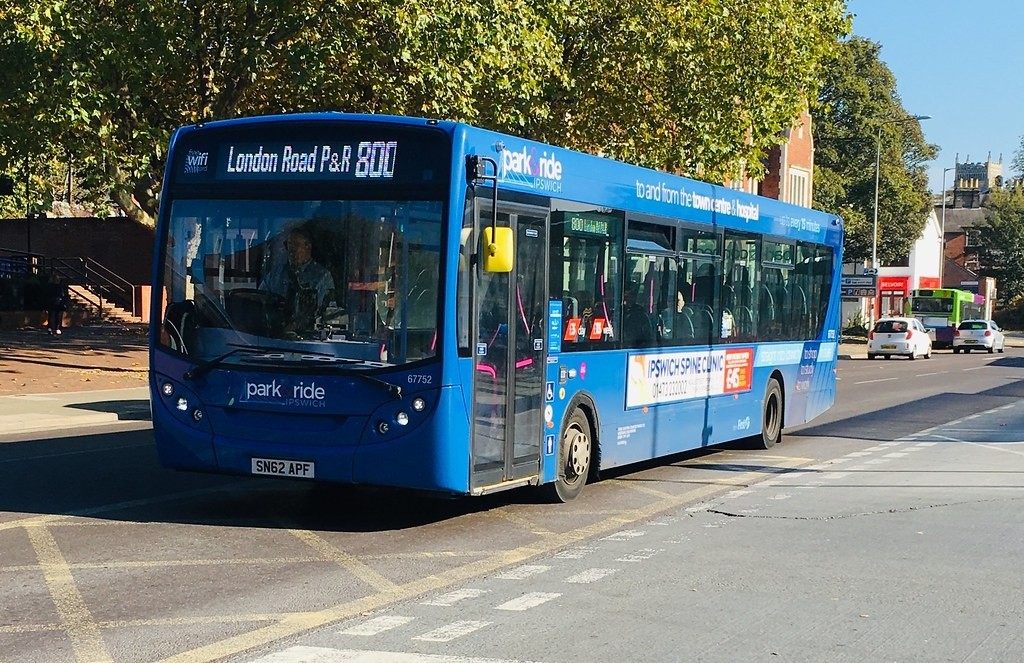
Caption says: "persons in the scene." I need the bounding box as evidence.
[571,286,693,343]
[244,231,336,340]
[44,275,66,335]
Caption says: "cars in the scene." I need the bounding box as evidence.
[867,318,932,361]
[952,320,1005,353]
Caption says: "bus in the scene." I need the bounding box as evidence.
[144,112,847,509]
[905,289,986,350]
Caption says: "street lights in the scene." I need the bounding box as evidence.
[939,167,956,290]
[872,114,933,269]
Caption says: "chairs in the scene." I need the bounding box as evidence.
[560,262,809,347]
